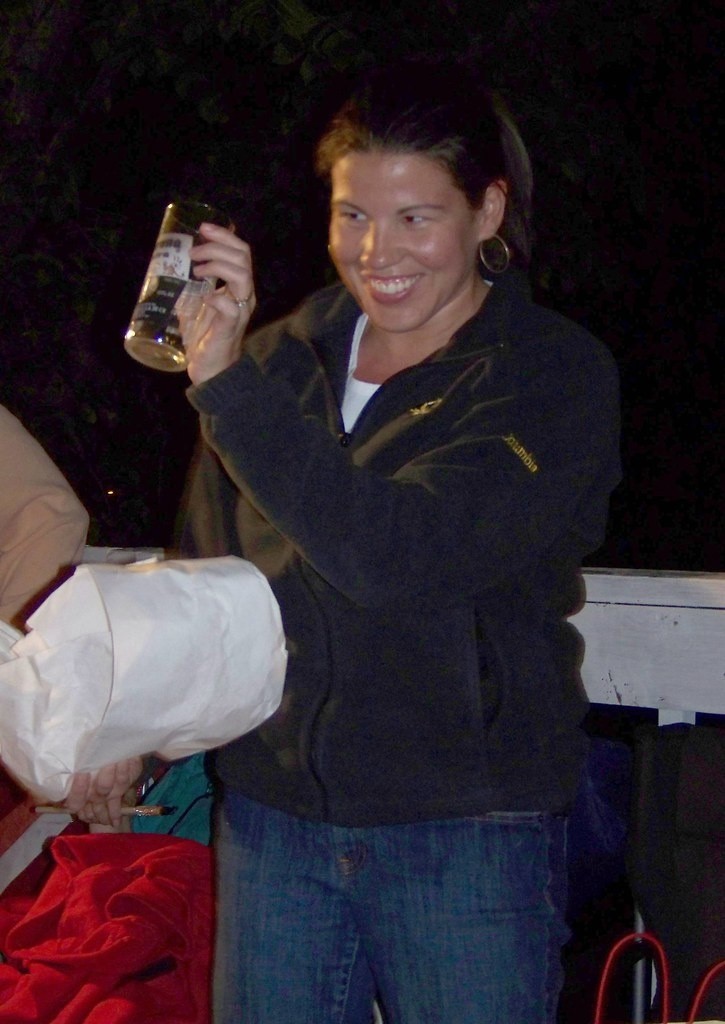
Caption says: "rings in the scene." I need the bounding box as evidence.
[227,285,255,307]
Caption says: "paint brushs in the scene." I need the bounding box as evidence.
[30,802,178,820]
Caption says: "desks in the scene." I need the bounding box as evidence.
[568,566,725,1024]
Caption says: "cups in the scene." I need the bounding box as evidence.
[123,199,237,374]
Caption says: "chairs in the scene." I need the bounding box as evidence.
[625,723,725,1024]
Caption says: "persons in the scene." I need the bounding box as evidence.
[0,403,214,845]
[172,88,623,1024]
